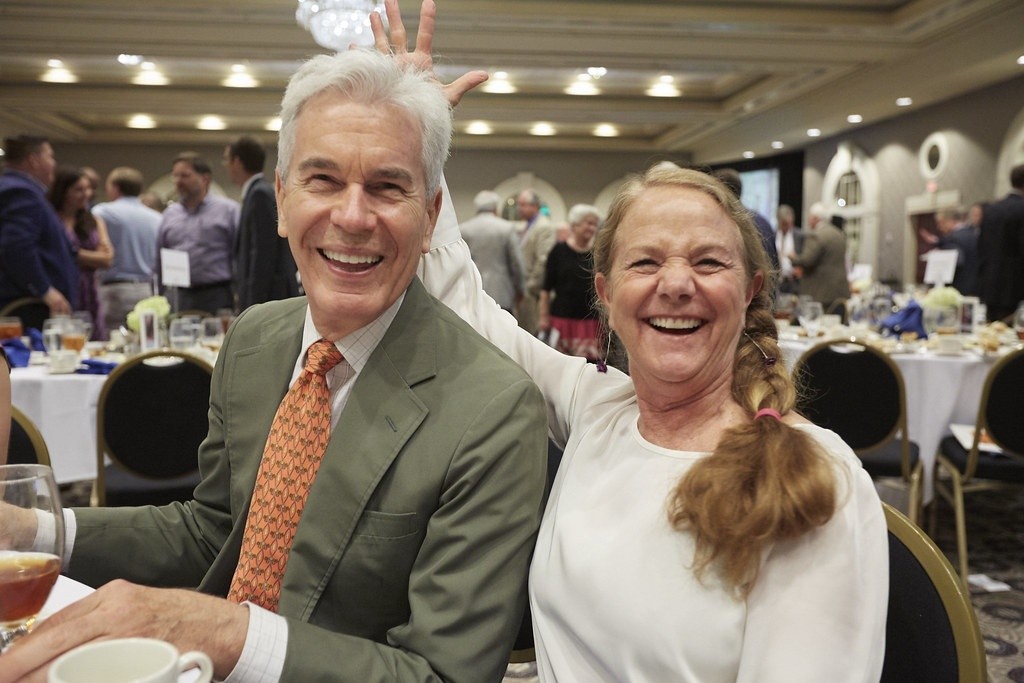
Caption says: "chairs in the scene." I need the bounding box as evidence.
[930,342,1024,604]
[89,350,213,507]
[5,405,52,509]
[791,335,925,528]
[880,500,988,683]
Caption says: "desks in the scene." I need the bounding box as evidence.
[777,321,1020,507]
[10,341,220,483]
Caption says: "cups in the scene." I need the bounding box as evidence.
[46,635,213,683]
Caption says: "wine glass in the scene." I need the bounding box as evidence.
[774,293,1024,355]
[0,308,237,372]
[0,463,66,656]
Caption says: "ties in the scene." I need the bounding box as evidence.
[226,337,344,610]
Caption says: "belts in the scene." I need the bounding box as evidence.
[102,277,153,285]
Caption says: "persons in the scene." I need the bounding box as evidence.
[0,135,852,363]
[0,52,549,682]
[349,0,890,683]
[917,163,1024,325]
